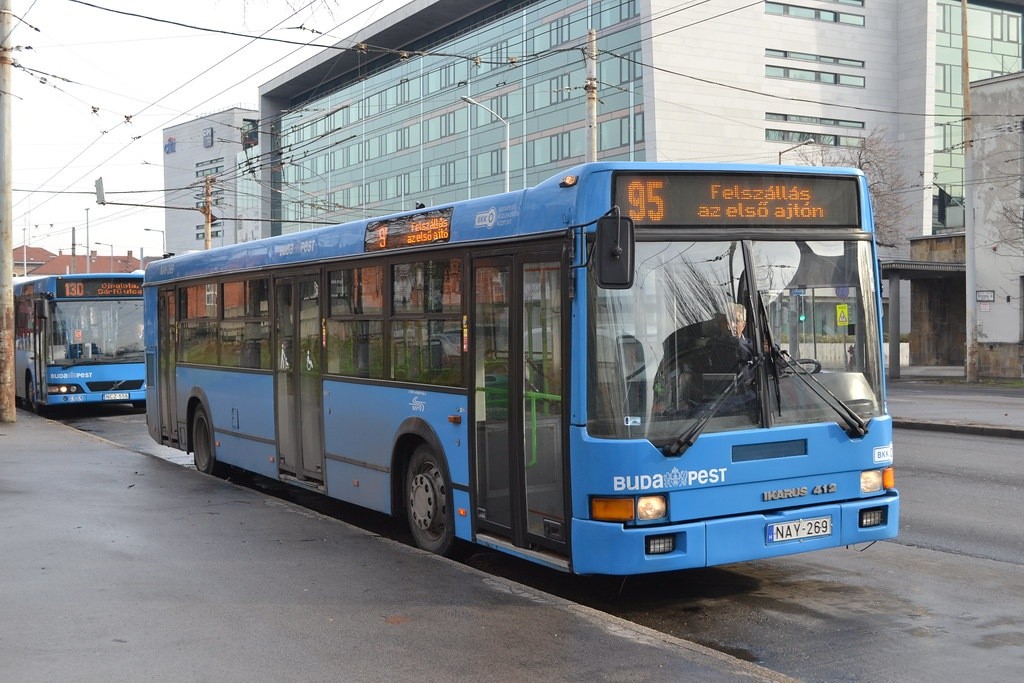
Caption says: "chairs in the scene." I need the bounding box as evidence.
[234,332,514,420]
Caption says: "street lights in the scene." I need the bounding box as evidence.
[460,95,510,192]
[85,208,91,273]
[144,228,165,254]
[778,138,815,164]
[22,228,27,277]
[94,242,113,273]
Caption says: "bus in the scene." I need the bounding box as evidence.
[12,269,146,418]
[141,161,901,577]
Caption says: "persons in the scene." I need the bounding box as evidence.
[674,301,773,419]
